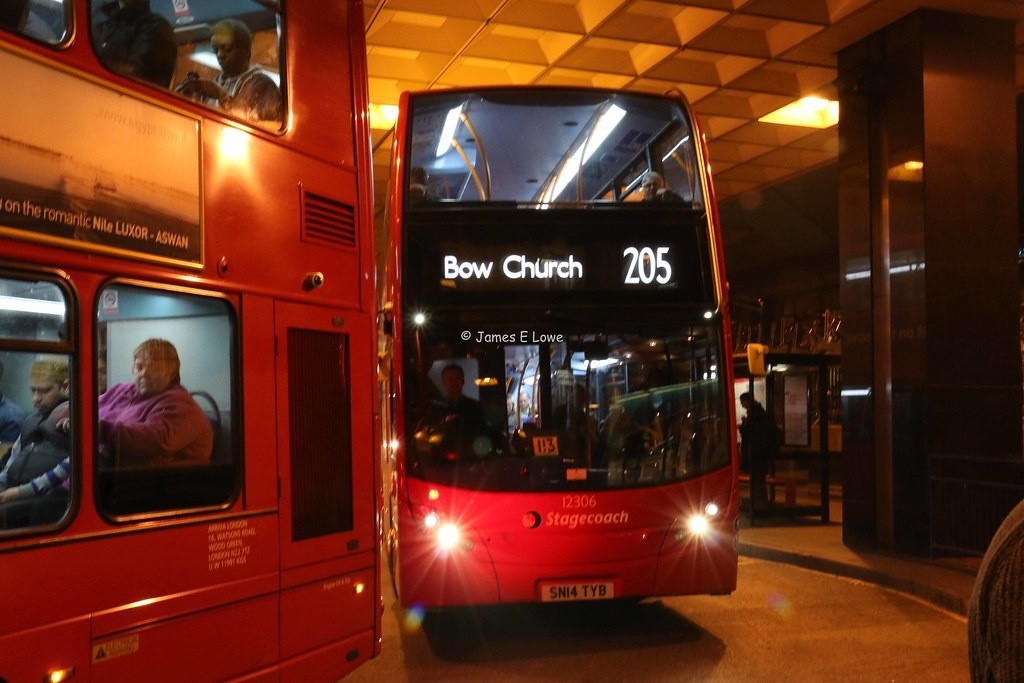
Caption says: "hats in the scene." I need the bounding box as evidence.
[29,359,69,383]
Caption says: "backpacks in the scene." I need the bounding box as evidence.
[768,423,784,449]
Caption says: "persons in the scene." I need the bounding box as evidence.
[416,363,484,455]
[0,340,213,527]
[641,171,683,202]
[92,0,179,89]
[411,167,437,202]
[968,498,1024,683]
[507,392,539,428]
[736,392,771,519]
[174,19,282,123]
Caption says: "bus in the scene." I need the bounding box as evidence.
[0,0,391,683]
[376,79,776,611]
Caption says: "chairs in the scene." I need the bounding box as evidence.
[189,391,221,428]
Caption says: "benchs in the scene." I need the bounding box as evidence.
[737,474,808,501]
